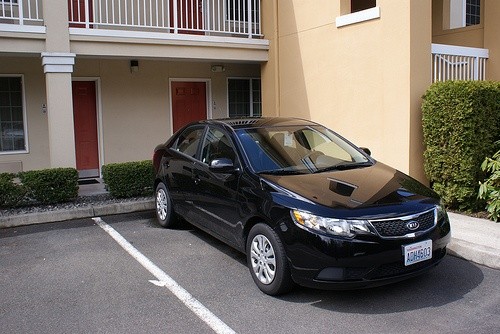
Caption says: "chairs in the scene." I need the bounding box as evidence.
[272,134,304,166]
[212,136,238,169]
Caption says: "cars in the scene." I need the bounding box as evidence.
[152,118,452,297]
